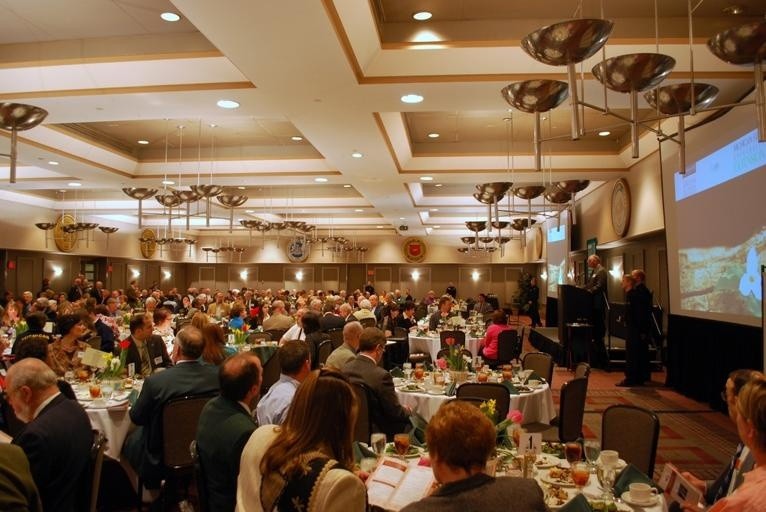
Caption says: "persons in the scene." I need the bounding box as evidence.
[2,253,766,512]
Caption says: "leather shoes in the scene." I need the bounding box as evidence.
[615,378,652,388]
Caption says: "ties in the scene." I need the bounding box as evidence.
[141,342,150,375]
[712,444,744,504]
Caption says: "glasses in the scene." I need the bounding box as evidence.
[721,392,737,402]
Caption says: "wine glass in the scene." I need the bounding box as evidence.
[583,438,601,465]
[401,359,519,386]
[370,432,386,457]
[601,452,618,483]
[564,442,582,464]
[392,432,411,457]
[86,384,113,406]
[571,462,590,493]
[416,311,486,336]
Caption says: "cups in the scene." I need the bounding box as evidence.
[627,482,658,502]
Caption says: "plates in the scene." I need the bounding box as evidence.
[517,452,560,468]
[621,492,659,508]
[539,471,573,487]
[380,441,420,458]
[543,489,573,509]
